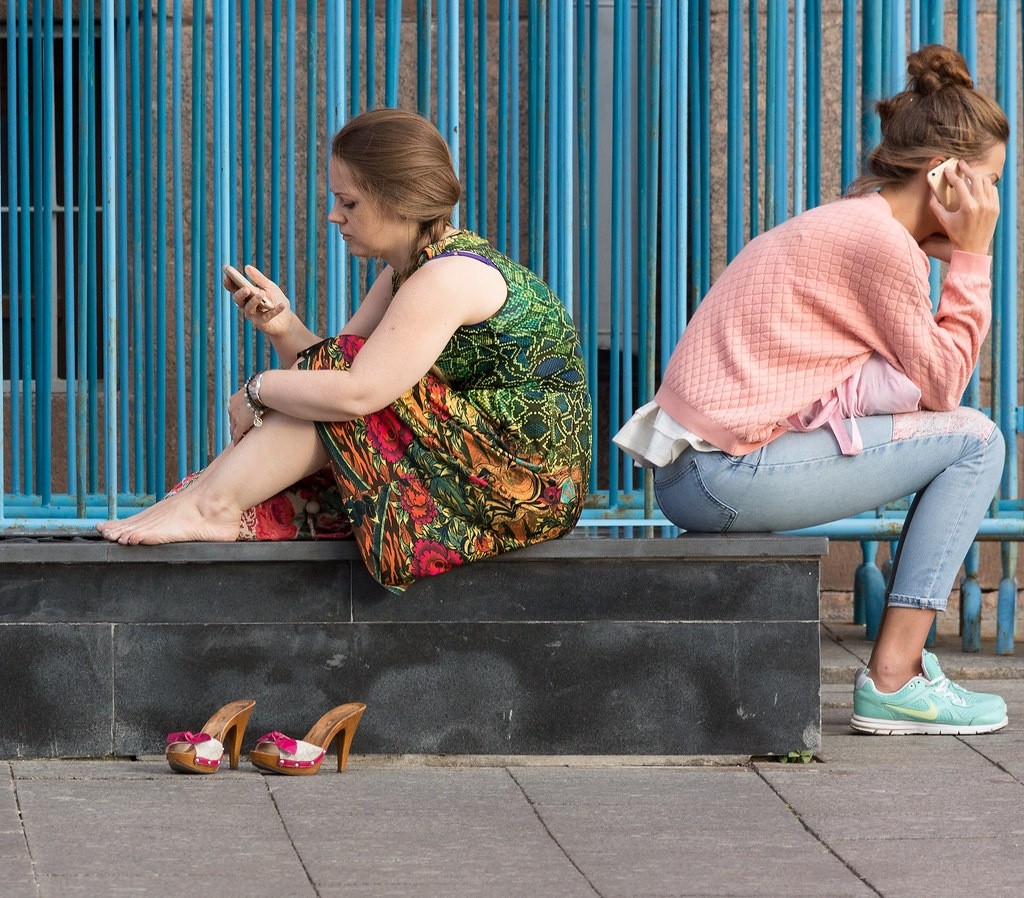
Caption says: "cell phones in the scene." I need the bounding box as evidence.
[926,157,974,212]
[222,264,275,310]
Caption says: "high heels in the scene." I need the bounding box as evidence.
[164,699,256,774]
[249,702,366,774]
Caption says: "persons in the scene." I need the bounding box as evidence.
[611,43,1010,735]
[92,107,592,596]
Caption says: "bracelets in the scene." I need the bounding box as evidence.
[243,371,270,426]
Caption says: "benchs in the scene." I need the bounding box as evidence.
[0,525,830,763]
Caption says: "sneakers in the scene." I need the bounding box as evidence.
[849,647,1008,737]
